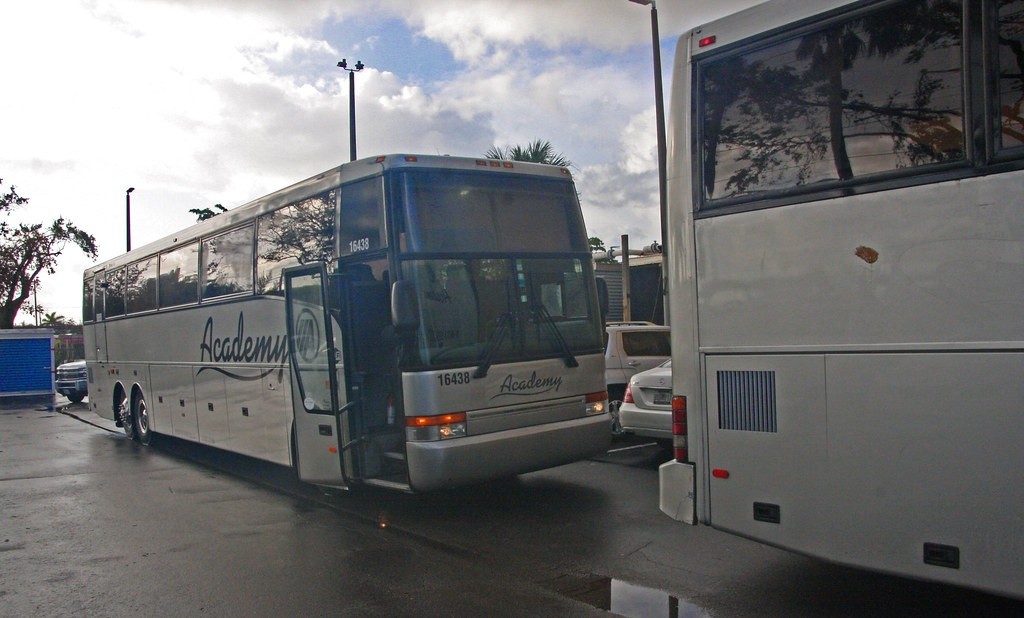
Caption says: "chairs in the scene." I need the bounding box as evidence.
[341,263,479,301]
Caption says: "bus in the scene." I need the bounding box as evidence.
[659,0,1024,597]
[82,154,613,498]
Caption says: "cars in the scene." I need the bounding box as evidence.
[605,326,672,441]
[618,357,674,447]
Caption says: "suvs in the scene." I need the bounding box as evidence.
[54,361,88,402]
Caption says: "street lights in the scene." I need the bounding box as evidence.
[126,187,135,252]
[337,59,364,161]
[629,0,670,325]
